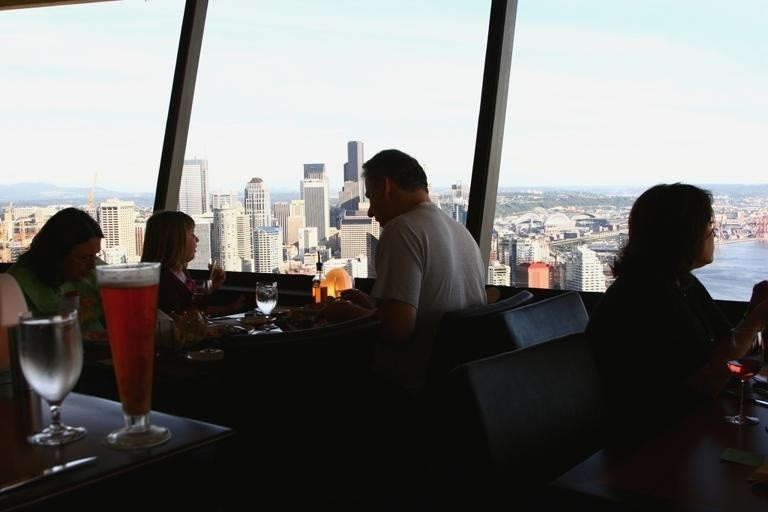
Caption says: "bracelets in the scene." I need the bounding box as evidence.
[730,327,749,358]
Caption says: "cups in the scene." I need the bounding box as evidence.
[95,260,171,451]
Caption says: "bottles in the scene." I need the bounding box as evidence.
[311,261,330,305]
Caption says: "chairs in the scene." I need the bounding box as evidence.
[490,292,595,348]
[350,295,533,444]
[447,335,610,509]
[232,316,378,466]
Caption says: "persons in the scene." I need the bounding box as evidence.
[141,211,248,373]
[582,185,767,451]
[7,207,185,403]
[315,150,488,412]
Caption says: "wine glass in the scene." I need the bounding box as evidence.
[190,277,214,326]
[18,307,88,448]
[721,326,766,427]
[254,280,280,330]
